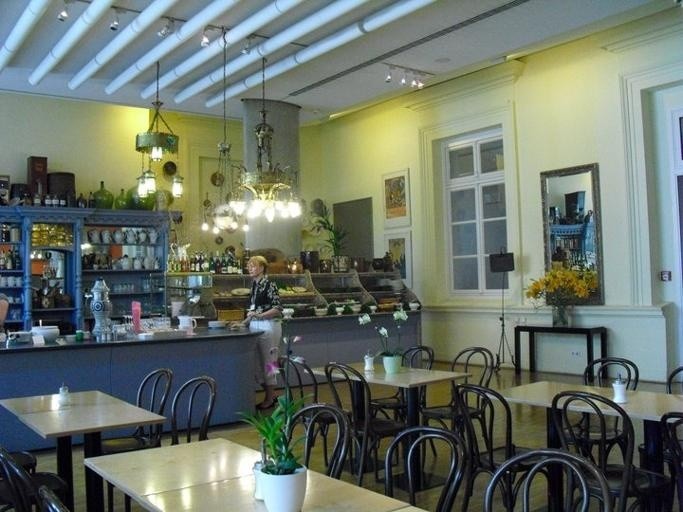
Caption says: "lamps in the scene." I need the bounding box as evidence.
[135,61,183,200]
[51,0,250,235]
[228,57,304,224]
[488,247,521,374]
[378,61,436,90]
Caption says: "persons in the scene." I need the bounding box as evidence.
[0,293,10,329]
[229,255,283,410]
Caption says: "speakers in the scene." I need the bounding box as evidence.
[490,253,514,272]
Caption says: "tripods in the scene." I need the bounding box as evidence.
[495,273,518,373]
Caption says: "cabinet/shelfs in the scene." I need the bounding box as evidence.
[166,268,423,394]
[0,207,184,331]
[550,223,595,271]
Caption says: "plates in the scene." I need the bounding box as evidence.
[230,287,251,296]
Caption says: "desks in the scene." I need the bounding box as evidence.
[1,388,167,512]
[514,324,608,383]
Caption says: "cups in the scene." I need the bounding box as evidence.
[0,333,5,342]
[363,358,373,370]
[76,329,83,340]
[300,251,318,273]
[112,282,135,292]
[152,316,170,332]
[177,315,197,335]
[351,256,365,272]
[7,296,20,303]
[0,276,22,287]
[320,260,331,272]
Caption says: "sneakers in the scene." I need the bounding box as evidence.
[255,395,279,411]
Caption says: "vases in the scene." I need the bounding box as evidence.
[552,299,568,328]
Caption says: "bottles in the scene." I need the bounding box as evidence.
[154,188,174,211]
[167,248,251,274]
[93,180,113,210]
[23,191,95,208]
[126,178,154,210]
[611,377,627,403]
[252,460,274,500]
[82,250,112,270]
[0,223,20,244]
[58,382,71,406]
[0,250,22,270]
[115,189,128,210]
[89,276,112,333]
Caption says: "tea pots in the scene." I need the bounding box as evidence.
[119,254,161,271]
[286,259,303,273]
[87,228,159,246]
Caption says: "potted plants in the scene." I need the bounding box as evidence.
[306,199,350,272]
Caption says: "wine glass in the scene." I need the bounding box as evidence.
[122,314,135,333]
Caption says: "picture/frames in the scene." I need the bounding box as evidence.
[380,166,411,229]
[383,229,413,290]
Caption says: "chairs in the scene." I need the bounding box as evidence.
[0,447,70,512]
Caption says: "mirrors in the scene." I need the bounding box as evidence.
[538,163,606,306]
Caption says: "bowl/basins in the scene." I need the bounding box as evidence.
[335,307,343,312]
[369,306,376,311]
[10,332,30,342]
[207,320,225,327]
[408,302,419,310]
[31,326,60,342]
[315,309,327,315]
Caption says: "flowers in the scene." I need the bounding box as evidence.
[521,269,592,325]
[233,315,314,475]
[552,260,598,291]
[358,308,408,358]
